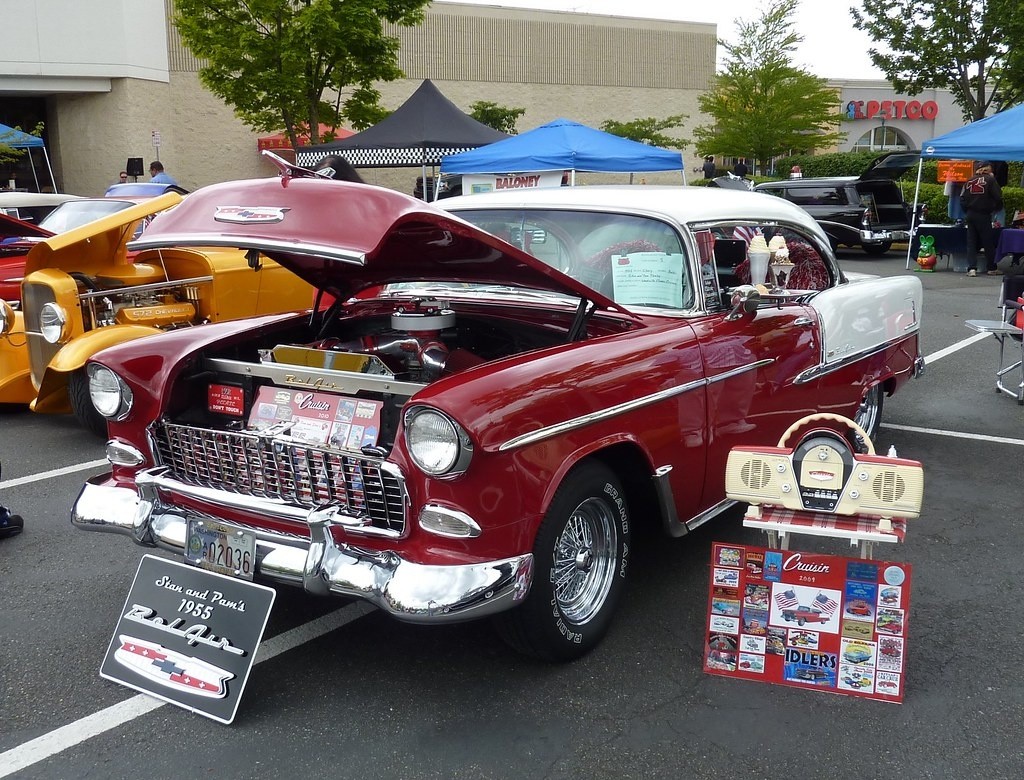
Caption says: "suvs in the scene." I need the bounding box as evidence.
[706,150,928,254]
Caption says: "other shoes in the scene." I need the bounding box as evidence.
[988,269,1003,274]
[0,515,24,540]
[966,269,976,276]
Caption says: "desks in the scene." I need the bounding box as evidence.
[743,502,907,561]
[910,226,1024,273]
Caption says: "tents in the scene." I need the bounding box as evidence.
[257,118,357,153]
[295,78,513,202]
[905,98,1023,271]
[433,118,685,202]
[0,122,59,192]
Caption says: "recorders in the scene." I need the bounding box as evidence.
[725,413,924,520]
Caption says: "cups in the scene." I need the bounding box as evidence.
[748,253,771,286]
[9,179,15,189]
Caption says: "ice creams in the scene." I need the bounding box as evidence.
[747,232,795,290]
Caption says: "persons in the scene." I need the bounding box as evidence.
[734,158,749,176]
[703,155,716,177]
[148,160,177,184]
[792,162,800,174]
[116,170,129,185]
[313,155,366,184]
[960,160,1004,277]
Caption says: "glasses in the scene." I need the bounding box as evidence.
[120,176,128,178]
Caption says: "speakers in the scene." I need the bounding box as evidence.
[127,157,144,176]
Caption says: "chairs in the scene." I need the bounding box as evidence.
[579,222,682,307]
[713,268,745,288]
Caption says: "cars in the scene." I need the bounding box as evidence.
[0,184,317,438]
[71,149,925,660]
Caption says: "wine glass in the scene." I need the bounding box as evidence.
[769,252,789,294]
[772,263,795,295]
